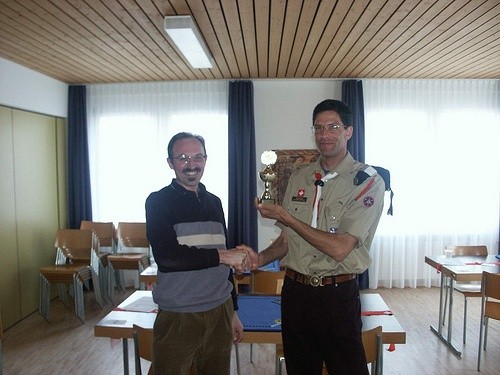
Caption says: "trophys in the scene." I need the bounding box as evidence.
[257,150,278,206]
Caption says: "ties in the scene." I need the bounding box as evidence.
[311,171,338,229]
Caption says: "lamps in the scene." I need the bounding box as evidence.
[164,16,214,69]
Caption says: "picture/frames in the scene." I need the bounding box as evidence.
[271,149,321,206]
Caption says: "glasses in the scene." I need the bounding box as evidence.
[311,123,347,135]
[170,154,207,164]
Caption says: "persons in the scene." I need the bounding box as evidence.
[145,132,252,375]
[236,99,385,375]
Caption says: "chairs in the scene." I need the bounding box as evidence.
[443,246,500,371]
[40,220,150,324]
[250,270,383,375]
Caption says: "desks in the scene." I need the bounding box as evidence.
[424,255,500,356]
[140,259,287,284]
[95,290,406,375]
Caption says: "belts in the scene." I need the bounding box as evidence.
[283,265,356,287]
